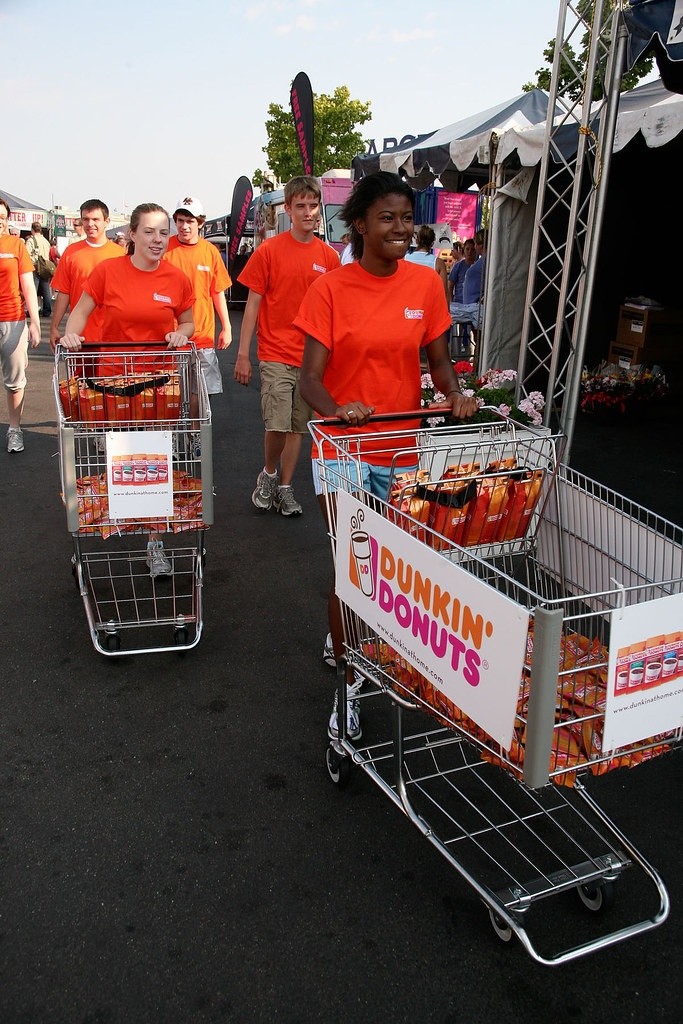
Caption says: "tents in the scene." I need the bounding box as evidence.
[349,81,592,383]
[481,78,683,394]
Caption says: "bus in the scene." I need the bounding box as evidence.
[198,213,255,311]
[251,168,368,266]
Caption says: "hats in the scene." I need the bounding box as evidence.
[175,196,205,218]
[11,229,18,235]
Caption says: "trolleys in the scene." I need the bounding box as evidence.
[50,339,214,664]
[305,399,683,968]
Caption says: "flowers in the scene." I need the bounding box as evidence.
[419,361,546,427]
[578,358,670,414]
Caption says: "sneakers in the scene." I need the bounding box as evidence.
[323,632,366,683]
[146,537,171,572]
[252,469,278,511]
[327,680,362,741]
[273,484,302,516]
[6,430,24,453]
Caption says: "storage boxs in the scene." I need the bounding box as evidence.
[609,341,673,367]
[616,306,676,347]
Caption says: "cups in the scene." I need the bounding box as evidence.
[616,654,683,691]
[114,469,167,481]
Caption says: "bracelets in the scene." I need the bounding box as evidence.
[446,389,465,400]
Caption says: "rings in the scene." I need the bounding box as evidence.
[348,410,354,415]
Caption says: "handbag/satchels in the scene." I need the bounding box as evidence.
[37,255,56,280]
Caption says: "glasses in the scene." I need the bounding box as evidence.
[74,224,82,226]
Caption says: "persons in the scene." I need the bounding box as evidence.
[68,218,87,246]
[405,224,451,314]
[60,203,197,579]
[0,200,42,453]
[234,176,343,519]
[296,172,483,743]
[50,198,129,354]
[25,222,62,319]
[112,232,127,247]
[446,228,488,377]
[168,194,233,395]
[338,230,354,266]
[10,220,25,244]
